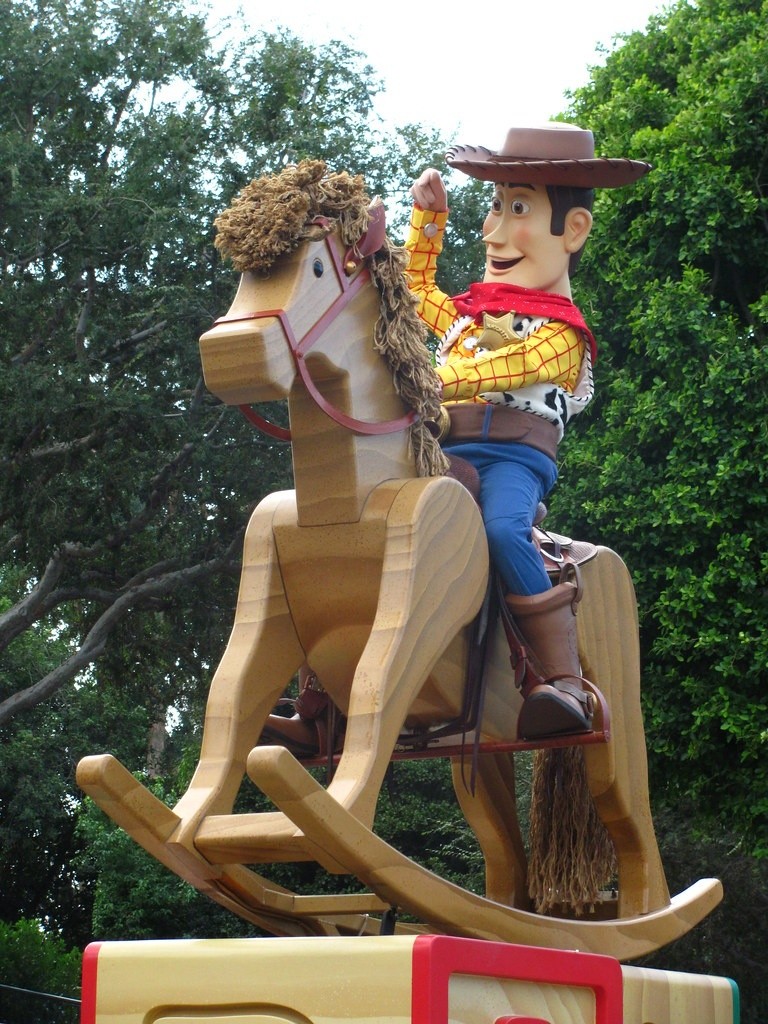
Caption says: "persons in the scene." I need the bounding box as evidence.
[258,121,653,757]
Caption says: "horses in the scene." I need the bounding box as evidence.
[171,161,673,920]
